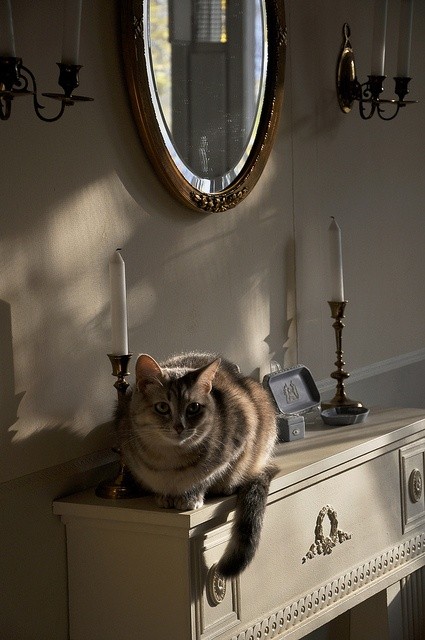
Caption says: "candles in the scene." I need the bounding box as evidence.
[62,1,83,62]
[371,2,388,76]
[397,3,411,77]
[1,0,18,52]
[328,217,345,304]
[108,248,130,356]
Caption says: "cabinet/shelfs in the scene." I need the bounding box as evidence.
[52,401,425,638]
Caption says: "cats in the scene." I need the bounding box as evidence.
[115,349,282,581]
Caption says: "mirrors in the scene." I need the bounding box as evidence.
[113,1,288,215]
